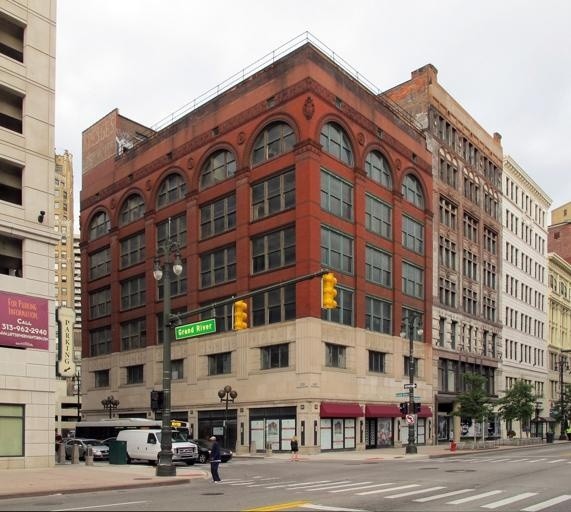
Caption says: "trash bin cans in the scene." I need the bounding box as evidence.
[546,432,554,443]
[110,441,127,464]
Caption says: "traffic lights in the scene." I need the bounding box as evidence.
[242,303,247,329]
[150,390,163,411]
[400,402,409,415]
[332,277,338,308]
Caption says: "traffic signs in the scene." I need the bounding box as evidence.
[175,319,217,340]
[414,396,420,400]
[404,383,418,389]
[396,392,410,397]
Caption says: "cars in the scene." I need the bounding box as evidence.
[189,439,233,464]
[55,438,73,448]
[102,436,116,446]
[67,430,75,438]
[66,438,110,460]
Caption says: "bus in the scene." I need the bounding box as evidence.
[75,417,193,442]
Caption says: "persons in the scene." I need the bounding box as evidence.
[207,436,222,485]
[289,435,299,462]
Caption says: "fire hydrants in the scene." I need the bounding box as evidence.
[451,441,457,450]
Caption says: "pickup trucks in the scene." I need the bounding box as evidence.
[117,430,199,466]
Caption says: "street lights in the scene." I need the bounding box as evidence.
[152,216,184,477]
[555,356,571,440]
[400,314,425,454]
[101,395,120,417]
[218,385,237,448]
